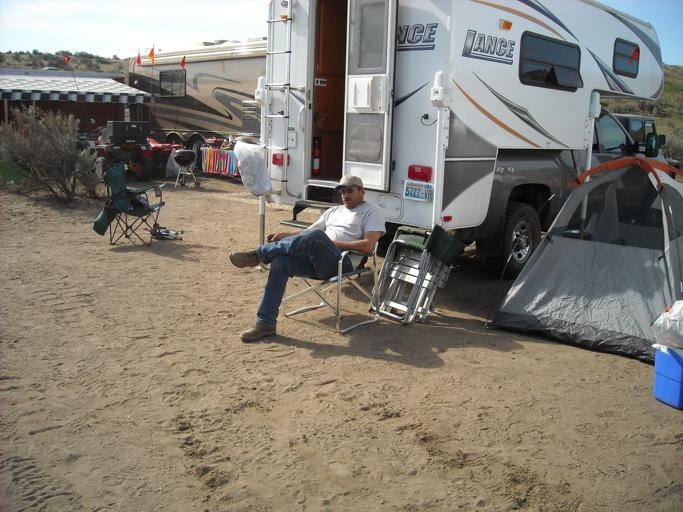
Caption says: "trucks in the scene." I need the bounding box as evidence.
[613,112,680,179]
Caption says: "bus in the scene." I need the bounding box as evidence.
[130,41,266,149]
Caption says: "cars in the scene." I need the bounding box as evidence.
[79,121,181,181]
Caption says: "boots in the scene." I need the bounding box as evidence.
[229,249,260,267]
[240,322,276,342]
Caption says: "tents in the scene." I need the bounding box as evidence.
[484,157,683,366]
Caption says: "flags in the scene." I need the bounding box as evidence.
[177,55,188,69]
[135,46,157,69]
[63,52,77,65]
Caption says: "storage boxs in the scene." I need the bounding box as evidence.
[653,342,682,411]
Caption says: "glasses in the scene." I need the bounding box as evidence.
[338,188,353,194]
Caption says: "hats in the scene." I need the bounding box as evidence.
[334,175,363,189]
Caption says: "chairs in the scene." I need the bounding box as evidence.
[282,230,379,333]
[104,161,166,247]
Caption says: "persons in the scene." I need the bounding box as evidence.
[228,175,386,343]
